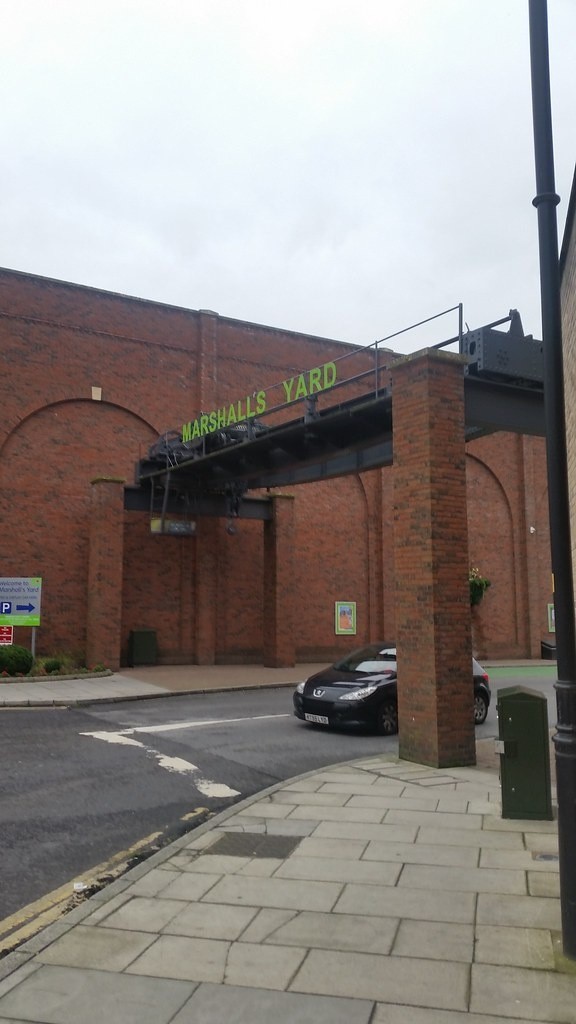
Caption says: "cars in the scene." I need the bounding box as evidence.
[292,640,492,739]
